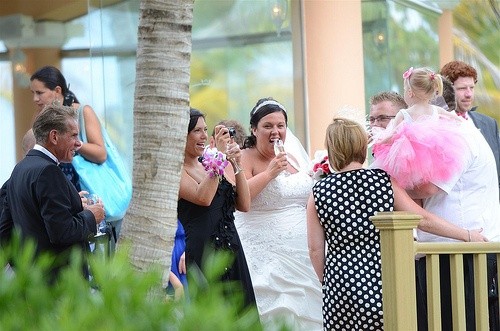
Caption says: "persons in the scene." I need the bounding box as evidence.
[177,108,263,331]
[372,67,471,208]
[440,61,500,188]
[223,98,328,331]
[369,92,408,129]
[30,66,132,258]
[307,118,488,331]
[412,76,500,331]
[7,105,105,284]
[167,219,189,317]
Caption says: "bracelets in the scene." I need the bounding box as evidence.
[467,229,472,242]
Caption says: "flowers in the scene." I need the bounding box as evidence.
[308,152,333,182]
[201,147,229,178]
[402,67,414,79]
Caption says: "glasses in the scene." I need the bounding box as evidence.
[368,114,396,123]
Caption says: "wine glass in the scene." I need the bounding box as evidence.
[274,140,292,177]
[214,139,245,175]
[87,194,106,238]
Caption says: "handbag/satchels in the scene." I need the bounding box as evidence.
[71,104,132,222]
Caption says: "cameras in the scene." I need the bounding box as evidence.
[223,127,236,137]
